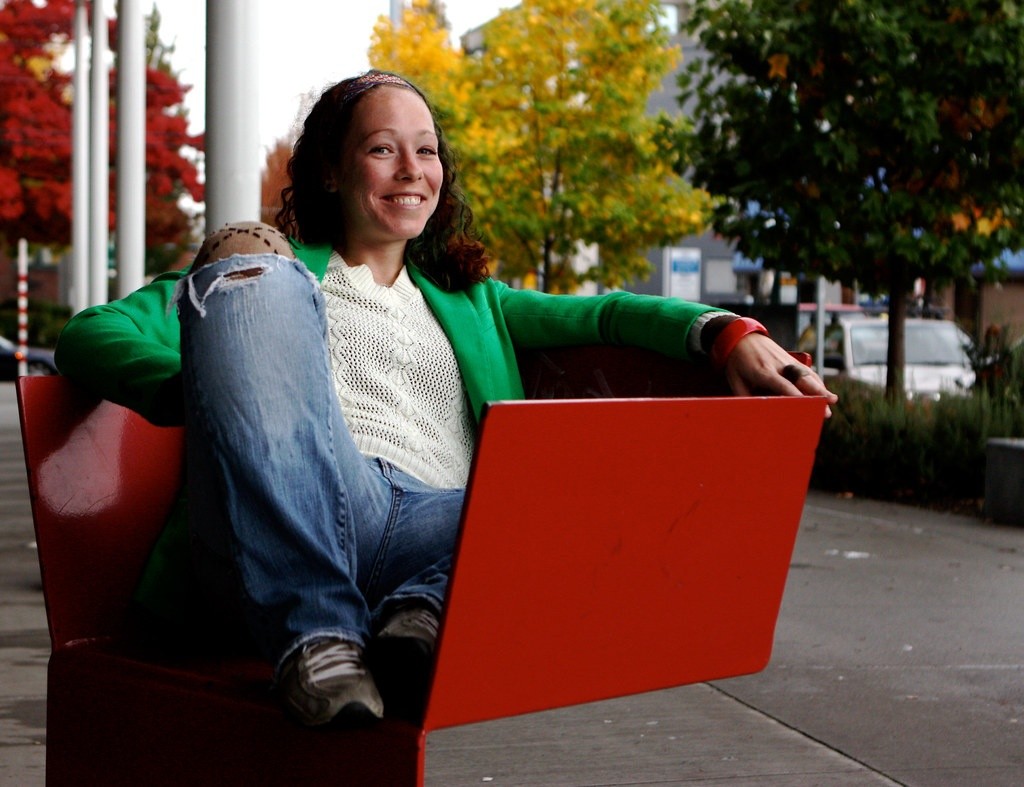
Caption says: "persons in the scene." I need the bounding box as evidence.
[54,74,838,731]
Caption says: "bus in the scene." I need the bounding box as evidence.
[699,295,956,351]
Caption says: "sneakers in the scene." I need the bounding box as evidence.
[278,636,385,737]
[371,597,445,718]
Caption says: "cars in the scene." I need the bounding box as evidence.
[14,263,155,310]
[813,319,977,401]
[0,335,57,383]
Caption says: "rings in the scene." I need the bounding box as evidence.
[781,365,809,386]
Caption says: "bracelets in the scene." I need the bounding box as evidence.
[711,317,770,364]
